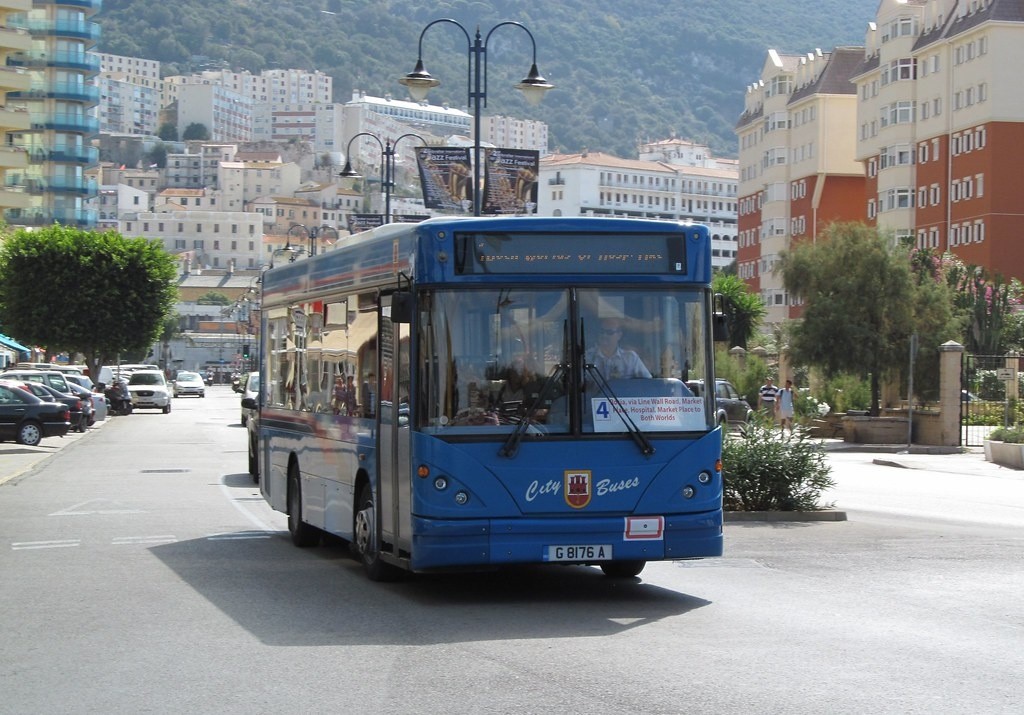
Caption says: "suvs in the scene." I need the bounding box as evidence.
[126,370,172,414]
[685,377,754,427]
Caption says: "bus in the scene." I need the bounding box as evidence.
[256,215,728,585]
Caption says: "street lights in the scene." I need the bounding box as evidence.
[226,262,273,374]
[281,224,342,259]
[335,131,431,225]
[396,16,556,218]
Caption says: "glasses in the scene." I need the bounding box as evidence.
[599,328,619,336]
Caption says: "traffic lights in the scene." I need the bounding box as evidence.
[242,344,249,358]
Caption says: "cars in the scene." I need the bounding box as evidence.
[237,372,260,427]
[171,372,206,397]
[0,362,161,430]
[200,371,211,384]
[0,383,71,446]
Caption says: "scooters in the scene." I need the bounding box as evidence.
[104,380,133,416]
[208,375,213,386]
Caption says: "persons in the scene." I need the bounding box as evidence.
[758,377,799,439]
[331,372,376,417]
[231,369,242,381]
[206,369,214,376]
[585,317,652,378]
[496,359,534,404]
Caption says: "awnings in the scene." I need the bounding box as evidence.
[0,334,31,354]
[307,311,377,362]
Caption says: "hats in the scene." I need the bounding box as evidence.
[766,377,774,381]
